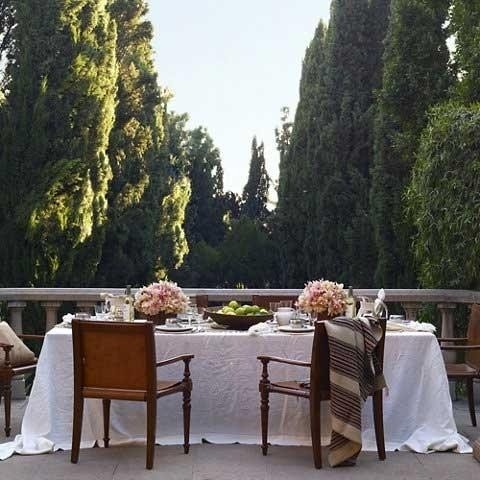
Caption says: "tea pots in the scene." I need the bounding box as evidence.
[354,298,389,325]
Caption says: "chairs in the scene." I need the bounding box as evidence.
[436,302,480,427]
[256,314,388,469]
[0,333,45,437]
[71,319,195,470]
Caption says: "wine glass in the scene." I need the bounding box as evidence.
[182,303,205,333]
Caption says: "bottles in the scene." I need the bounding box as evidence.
[122,284,136,322]
[345,286,357,319]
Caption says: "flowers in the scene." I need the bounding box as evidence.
[293,277,354,317]
[132,278,191,317]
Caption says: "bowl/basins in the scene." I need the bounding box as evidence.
[203,305,272,331]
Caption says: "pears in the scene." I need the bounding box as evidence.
[221,301,267,317]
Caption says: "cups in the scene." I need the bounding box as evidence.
[389,314,406,326]
[290,318,307,329]
[269,300,295,326]
[94,305,104,318]
[165,318,181,328]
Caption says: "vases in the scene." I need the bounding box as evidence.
[136,309,177,326]
[317,310,346,321]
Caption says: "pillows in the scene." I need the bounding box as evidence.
[0,321,35,367]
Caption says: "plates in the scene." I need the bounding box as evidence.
[279,325,316,332]
[155,324,193,332]
